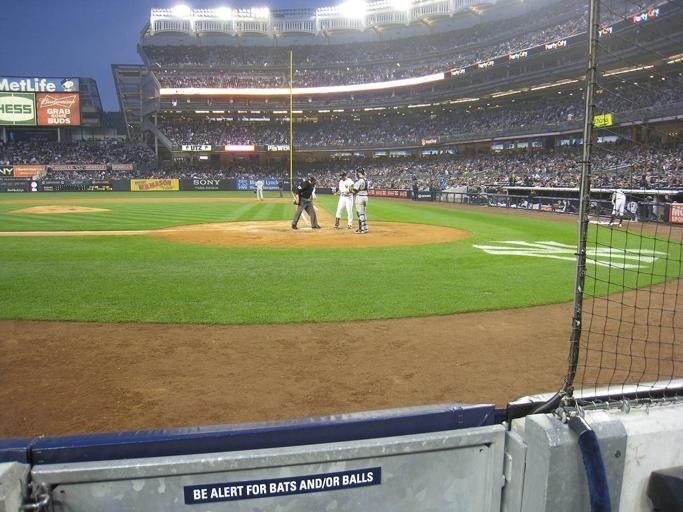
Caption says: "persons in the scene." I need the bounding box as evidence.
[332,171,354,229]
[254,177,264,201]
[2,1,683,192]
[291,177,321,230]
[608,190,627,228]
[350,166,368,234]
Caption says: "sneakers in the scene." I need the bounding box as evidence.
[334,223,340,228]
[312,225,320,229]
[292,224,298,229]
[355,228,368,233]
[348,225,352,229]
